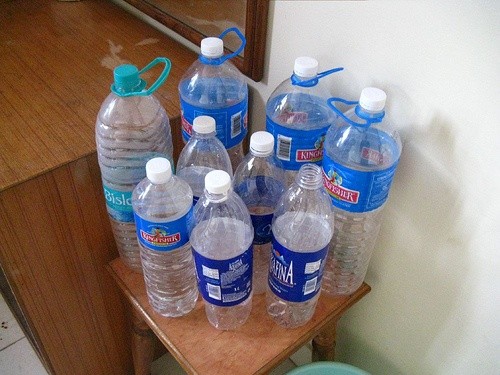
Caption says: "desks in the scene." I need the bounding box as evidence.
[106,258,371,375]
[0,0,250,375]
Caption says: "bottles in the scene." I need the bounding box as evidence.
[188,170,253,332]
[131,156,199,319]
[232,129,289,296]
[265,55,344,189]
[94,56,175,275]
[173,114,234,206]
[178,24,248,198]
[263,163,336,329]
[321,86,403,297]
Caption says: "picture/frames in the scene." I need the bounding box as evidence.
[124,0,269,82]
